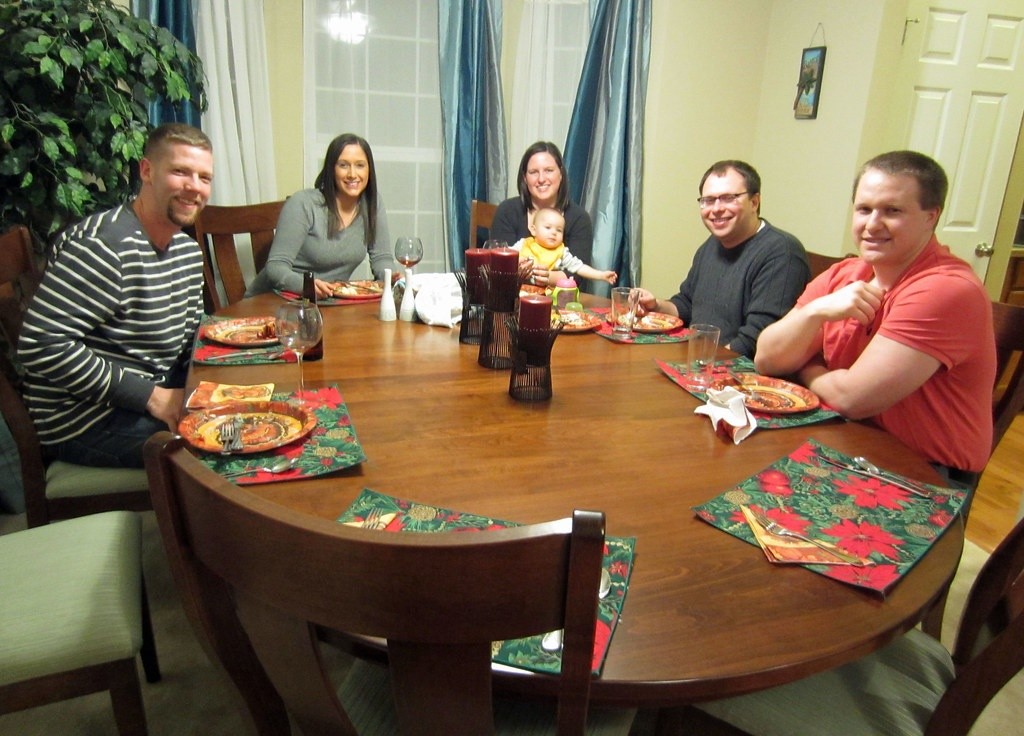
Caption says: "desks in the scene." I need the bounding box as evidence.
[176,280,964,736]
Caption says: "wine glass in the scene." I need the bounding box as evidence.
[393,238,424,278]
[274,301,323,398]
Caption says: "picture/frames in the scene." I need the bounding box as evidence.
[794,46,827,120]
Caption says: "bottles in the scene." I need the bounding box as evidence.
[298,270,324,360]
[381,269,415,321]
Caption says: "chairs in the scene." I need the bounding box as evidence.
[0,222,154,530]
[143,431,638,736]
[0,511,162,736]
[666,252,1024,736]
[190,193,290,314]
[469,200,498,249]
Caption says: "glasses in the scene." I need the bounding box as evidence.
[698,192,748,208]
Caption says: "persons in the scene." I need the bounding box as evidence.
[242,132,404,301]
[489,141,593,288]
[18,123,213,468]
[627,160,811,356]
[754,151,996,487]
[509,208,618,285]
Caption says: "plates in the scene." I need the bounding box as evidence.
[549,308,601,332]
[203,315,302,346]
[604,309,683,333]
[707,375,822,415]
[179,401,318,455]
[327,280,385,299]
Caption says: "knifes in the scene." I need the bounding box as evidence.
[203,349,272,359]
[817,455,931,500]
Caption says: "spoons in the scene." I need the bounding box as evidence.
[854,456,932,495]
[220,457,300,478]
[229,416,244,449]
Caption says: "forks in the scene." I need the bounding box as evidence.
[727,370,770,405]
[221,423,234,454]
[755,513,864,568]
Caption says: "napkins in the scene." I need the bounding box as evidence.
[693,385,758,446]
[186,380,274,409]
[739,503,877,567]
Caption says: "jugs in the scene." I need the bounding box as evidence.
[461,248,566,403]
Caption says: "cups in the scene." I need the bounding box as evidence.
[686,322,721,390]
[609,286,638,340]
[554,280,580,309]
